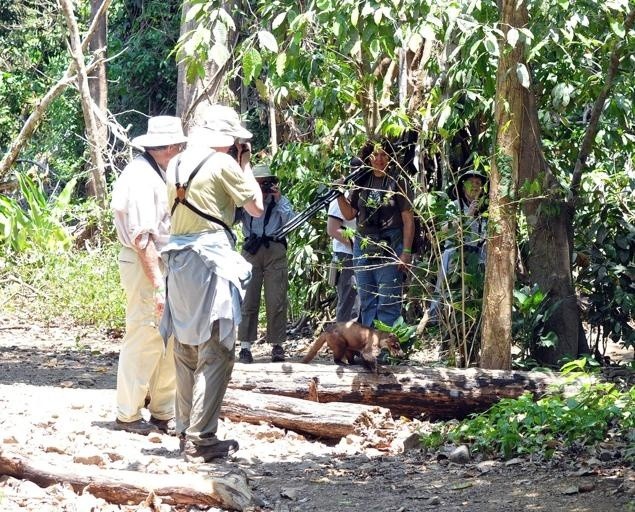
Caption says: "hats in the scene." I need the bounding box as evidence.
[457,170,487,192]
[190,105,254,148]
[133,116,187,147]
[251,163,276,179]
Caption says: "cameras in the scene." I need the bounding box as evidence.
[228,144,247,159]
[261,178,279,194]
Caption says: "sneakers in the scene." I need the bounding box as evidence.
[180,434,239,463]
[115,417,159,434]
[149,416,177,434]
[239,348,253,363]
[271,343,285,361]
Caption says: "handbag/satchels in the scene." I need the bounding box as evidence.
[328,261,339,286]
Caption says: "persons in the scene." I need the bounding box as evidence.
[427,171,491,366]
[333,136,416,365]
[111,115,188,437]
[325,187,362,323]
[161,102,265,461]
[239,164,297,364]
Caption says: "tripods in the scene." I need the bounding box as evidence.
[269,140,410,242]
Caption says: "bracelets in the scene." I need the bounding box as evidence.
[402,241,413,247]
[402,247,412,254]
